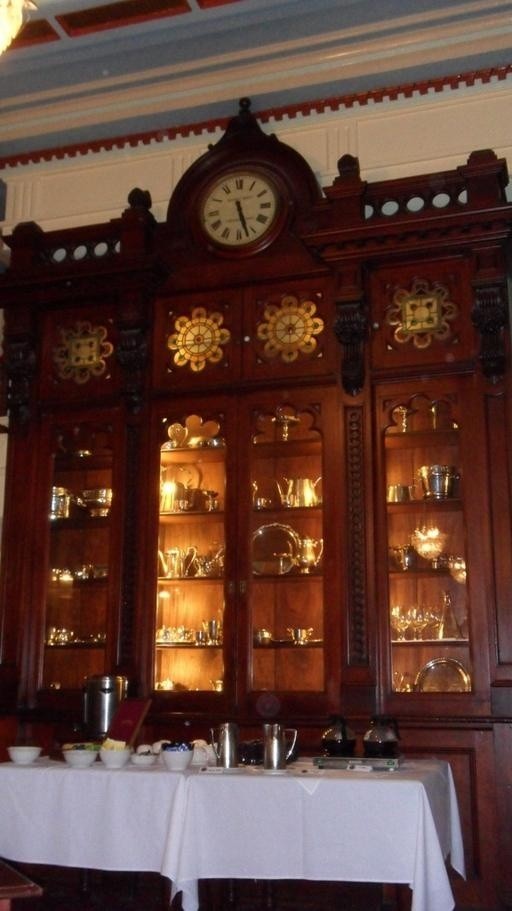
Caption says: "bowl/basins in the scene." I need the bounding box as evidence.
[208,438,223,447]
[7,745,43,765]
[198,560,219,577]
[62,741,218,772]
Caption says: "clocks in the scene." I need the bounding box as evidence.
[197,167,290,256]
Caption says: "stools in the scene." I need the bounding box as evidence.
[0,859,43,911]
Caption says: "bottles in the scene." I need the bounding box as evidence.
[387,484,411,502]
[279,420,290,441]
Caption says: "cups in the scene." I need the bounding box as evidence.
[390,544,417,571]
[431,400,451,431]
[258,631,271,644]
[254,495,272,511]
[50,485,69,518]
[210,679,223,691]
[198,494,212,512]
[162,680,174,690]
[287,627,313,645]
[398,409,409,432]
[54,630,70,645]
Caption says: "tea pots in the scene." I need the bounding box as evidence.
[158,544,198,578]
[274,476,323,509]
[261,723,298,769]
[321,712,358,758]
[284,532,326,573]
[159,466,195,514]
[362,714,403,756]
[209,723,241,768]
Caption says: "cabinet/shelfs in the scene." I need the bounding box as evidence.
[0,94,512,723]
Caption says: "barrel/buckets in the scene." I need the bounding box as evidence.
[50,486,73,520]
[81,488,113,517]
[81,673,129,742]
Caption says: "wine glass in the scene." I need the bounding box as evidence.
[192,618,222,646]
[389,603,441,641]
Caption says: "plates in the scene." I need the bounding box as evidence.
[410,527,444,559]
[251,523,299,576]
[414,658,470,694]
